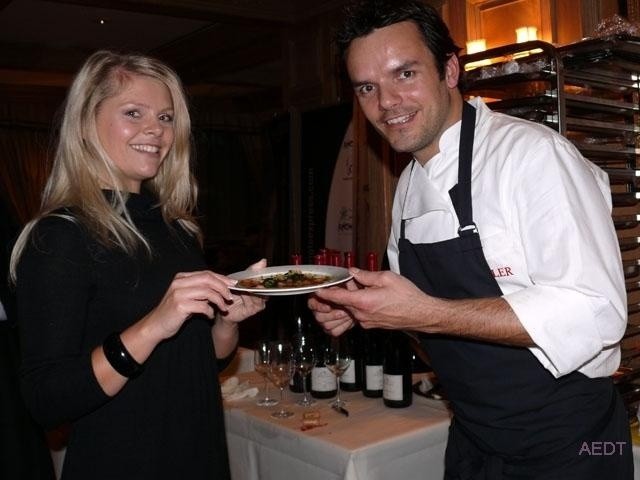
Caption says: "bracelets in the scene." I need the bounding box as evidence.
[101,332,142,378]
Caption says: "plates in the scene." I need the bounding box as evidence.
[223,264,355,298]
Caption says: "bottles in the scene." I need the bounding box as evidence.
[289,250,414,410]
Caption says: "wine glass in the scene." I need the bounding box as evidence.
[324,347,352,408]
[253,343,280,409]
[293,347,317,407]
[267,342,295,420]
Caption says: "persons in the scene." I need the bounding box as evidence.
[10,50,268,479]
[309,2,636,479]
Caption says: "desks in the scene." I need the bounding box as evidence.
[219,346,453,480]
